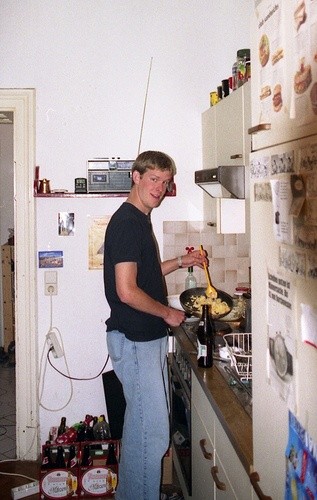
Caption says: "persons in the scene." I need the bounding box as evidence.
[103,151,208,500]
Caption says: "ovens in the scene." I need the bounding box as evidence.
[167,338,192,497]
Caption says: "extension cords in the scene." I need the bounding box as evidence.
[11,480,40,500]
[46,332,63,359]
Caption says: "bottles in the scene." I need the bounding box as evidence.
[66,471,72,496]
[231,49,251,91]
[42,415,118,469]
[106,468,112,494]
[185,266,197,290]
[233,287,248,307]
[39,179,50,194]
[196,305,213,369]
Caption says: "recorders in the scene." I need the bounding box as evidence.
[87,56,153,192]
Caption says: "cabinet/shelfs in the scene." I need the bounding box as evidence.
[192,368,259,500]
[201,79,252,235]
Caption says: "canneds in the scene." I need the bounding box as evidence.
[209,77,232,106]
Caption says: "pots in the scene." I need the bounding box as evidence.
[179,287,233,319]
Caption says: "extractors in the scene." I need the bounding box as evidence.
[194,165,245,200]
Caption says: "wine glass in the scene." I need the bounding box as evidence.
[72,477,78,496]
[111,474,117,494]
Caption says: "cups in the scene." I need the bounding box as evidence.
[210,77,232,106]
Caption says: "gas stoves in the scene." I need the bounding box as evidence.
[171,321,244,354]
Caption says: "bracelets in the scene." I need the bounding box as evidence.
[178,256,183,268]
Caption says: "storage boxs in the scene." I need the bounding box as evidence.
[39,439,120,500]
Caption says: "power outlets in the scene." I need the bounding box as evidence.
[45,284,57,295]
